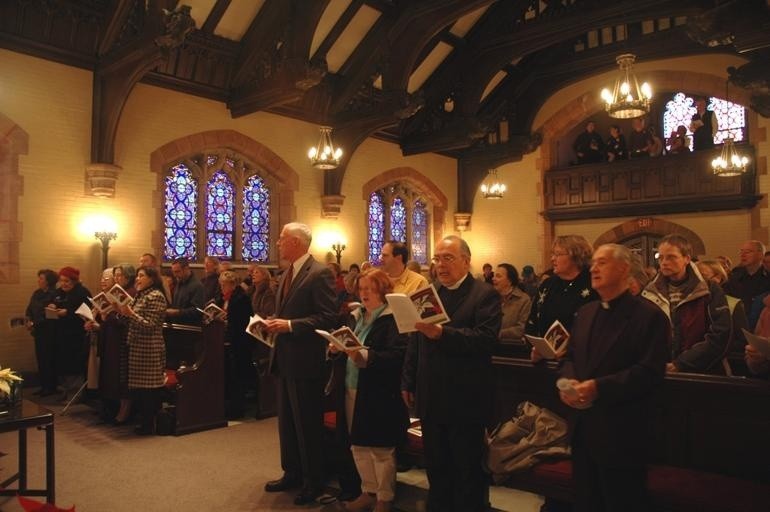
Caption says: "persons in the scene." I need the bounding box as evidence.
[574,96,718,165]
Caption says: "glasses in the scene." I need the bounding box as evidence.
[430,255,466,265]
[550,251,571,257]
[701,245,762,288]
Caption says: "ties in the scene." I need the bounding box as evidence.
[282,262,294,303]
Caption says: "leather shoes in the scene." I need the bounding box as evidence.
[264,471,303,492]
[294,484,328,505]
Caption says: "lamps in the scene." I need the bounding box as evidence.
[708,77,749,178]
[308,126,343,170]
[481,126,507,200]
[601,20,653,120]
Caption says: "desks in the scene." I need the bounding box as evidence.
[0,398,57,512]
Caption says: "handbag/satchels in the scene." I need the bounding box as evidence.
[155,409,177,436]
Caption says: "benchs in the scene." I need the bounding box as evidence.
[159,311,764,511]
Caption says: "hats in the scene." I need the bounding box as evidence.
[60,266,80,282]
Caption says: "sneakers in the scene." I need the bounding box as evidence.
[32,388,56,397]
[341,491,377,511]
[371,500,393,512]
[134,423,154,435]
[111,413,132,426]
